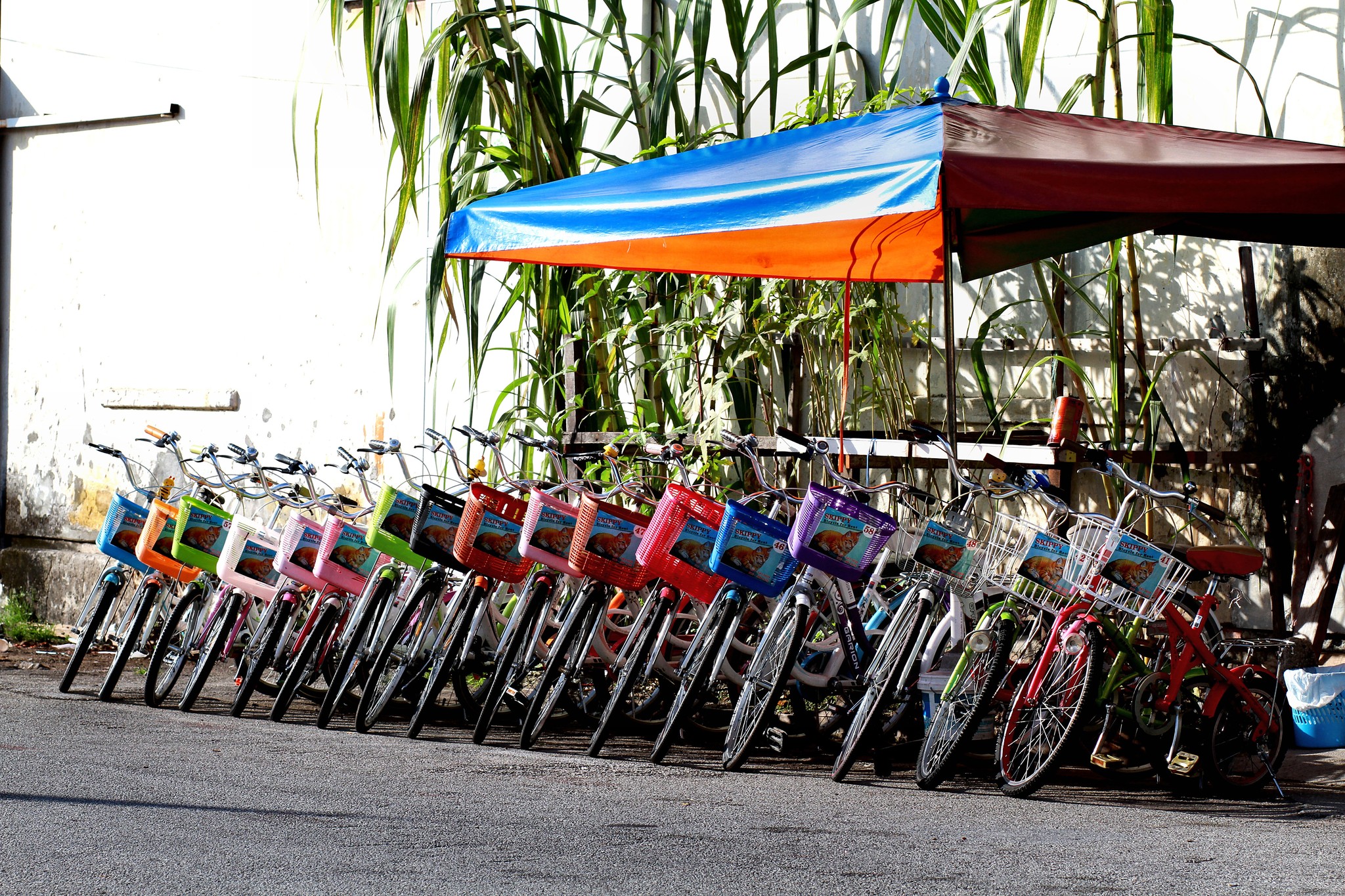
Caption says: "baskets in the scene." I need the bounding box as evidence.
[453,482,535,583]
[518,486,585,578]
[787,481,897,583]
[171,496,234,576]
[365,481,435,570]
[272,509,339,594]
[708,498,800,598]
[312,514,393,598]
[409,484,472,574]
[980,511,1088,616]
[1283,664,1344,748]
[135,497,203,582]
[1063,512,1194,621]
[567,491,658,591]
[635,483,734,605]
[95,492,156,575]
[216,514,289,601]
[894,492,1017,599]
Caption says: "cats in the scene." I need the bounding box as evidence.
[475,532,520,555]
[915,543,967,571]
[156,537,174,554]
[421,525,459,550]
[723,545,774,571]
[183,526,223,549]
[674,539,715,565]
[383,513,415,538]
[588,532,634,558]
[239,558,274,578]
[332,545,373,568]
[532,527,575,552]
[292,547,319,568]
[812,529,864,557]
[114,530,142,550]
[1023,555,1073,585]
[1105,559,1159,588]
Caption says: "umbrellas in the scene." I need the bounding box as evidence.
[447,77,1345,525]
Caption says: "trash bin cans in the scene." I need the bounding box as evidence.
[1284,665,1345,749]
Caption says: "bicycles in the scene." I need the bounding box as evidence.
[63,426,1296,801]
